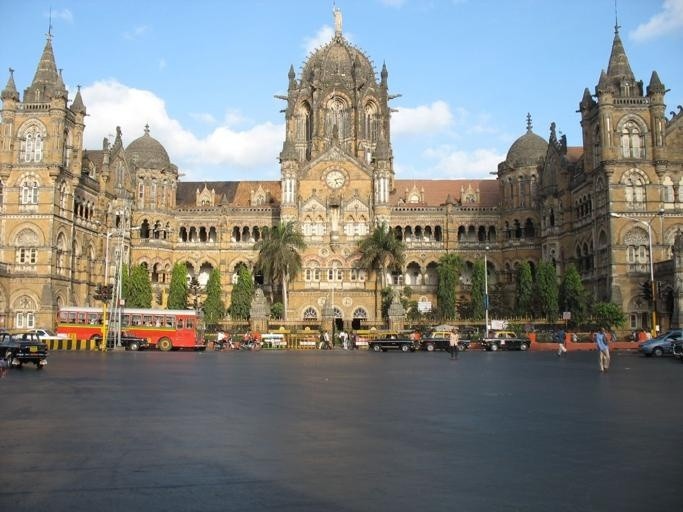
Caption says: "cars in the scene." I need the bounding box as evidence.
[640,329,683,359]
[369,334,416,352]
[94,330,149,351]
[0,329,66,368]
[420,332,471,351]
[481,331,531,351]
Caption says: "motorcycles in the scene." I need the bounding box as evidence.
[240,339,262,351]
[213,340,239,351]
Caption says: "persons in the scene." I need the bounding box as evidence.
[215,330,227,351]
[337,330,359,350]
[241,331,262,352]
[450,329,459,360]
[556,327,567,357]
[629,327,660,342]
[410,330,427,349]
[589,329,617,343]
[573,332,578,343]
[596,327,610,372]
[318,330,332,350]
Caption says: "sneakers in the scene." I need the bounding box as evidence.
[600,368,608,373]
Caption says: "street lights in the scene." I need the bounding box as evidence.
[105,226,141,285]
[484,246,490,340]
[609,209,665,338]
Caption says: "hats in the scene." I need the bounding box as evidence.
[453,330,457,333]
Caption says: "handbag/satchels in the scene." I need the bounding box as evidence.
[603,334,608,344]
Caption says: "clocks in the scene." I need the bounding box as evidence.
[325,170,346,189]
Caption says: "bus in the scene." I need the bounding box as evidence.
[56,306,207,351]
